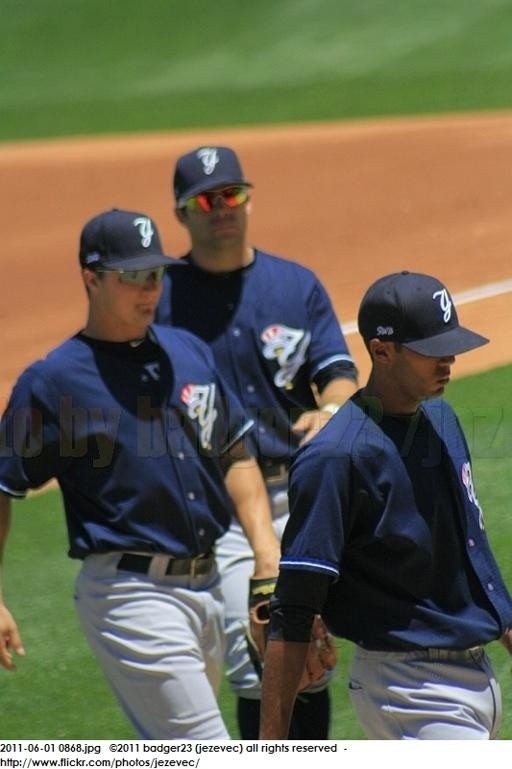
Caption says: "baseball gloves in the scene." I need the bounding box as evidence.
[247,578,336,693]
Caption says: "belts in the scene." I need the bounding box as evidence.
[264,461,292,477]
[117,551,217,581]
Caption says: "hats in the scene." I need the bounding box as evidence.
[79,208,188,269]
[358,272,489,357]
[173,146,252,210]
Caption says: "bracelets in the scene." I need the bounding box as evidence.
[320,404,341,413]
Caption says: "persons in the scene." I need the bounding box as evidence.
[152,144,360,739]
[1,206,336,739]
[257,270,509,738]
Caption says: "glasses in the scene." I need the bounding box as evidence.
[97,266,165,285]
[187,186,247,214]
